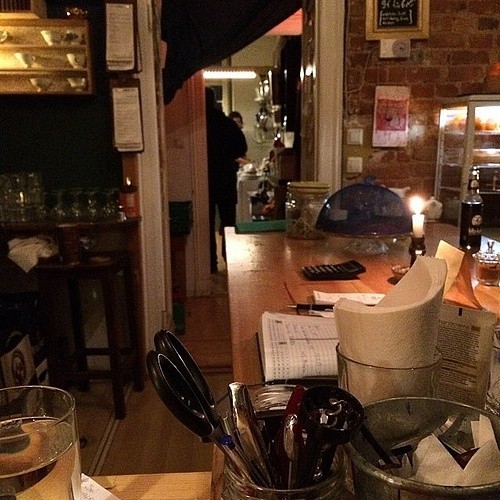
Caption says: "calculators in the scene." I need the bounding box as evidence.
[302,260,366,281]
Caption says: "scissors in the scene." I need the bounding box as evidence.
[146,329,241,474]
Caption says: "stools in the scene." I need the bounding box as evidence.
[36,249,142,419]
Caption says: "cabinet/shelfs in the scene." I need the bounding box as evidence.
[0,18,95,94]
[434,95,500,203]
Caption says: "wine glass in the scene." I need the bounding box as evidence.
[22,51,37,68]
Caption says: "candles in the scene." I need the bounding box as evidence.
[408,195,427,238]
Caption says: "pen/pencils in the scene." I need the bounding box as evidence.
[216,382,280,489]
[286,304,375,313]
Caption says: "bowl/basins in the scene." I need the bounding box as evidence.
[0,30,82,46]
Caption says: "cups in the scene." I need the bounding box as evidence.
[0,384,81,500]
[484,324,500,415]
[66,53,86,69]
[333,342,441,405]
[392,263,409,280]
[345,396,500,500]
[0,171,127,222]
[30,78,53,92]
[66,77,86,92]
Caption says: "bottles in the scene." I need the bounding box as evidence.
[120,176,139,218]
[408,233,427,268]
[210,384,354,500]
[459,165,484,255]
[471,240,500,287]
[285,180,331,240]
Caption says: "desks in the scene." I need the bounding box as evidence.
[223,223,500,500]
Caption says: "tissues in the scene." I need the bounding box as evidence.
[333,240,465,443]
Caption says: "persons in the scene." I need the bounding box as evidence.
[205,87,248,274]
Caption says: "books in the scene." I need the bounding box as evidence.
[257,311,340,382]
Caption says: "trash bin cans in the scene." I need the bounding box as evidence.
[169,201,194,304]
